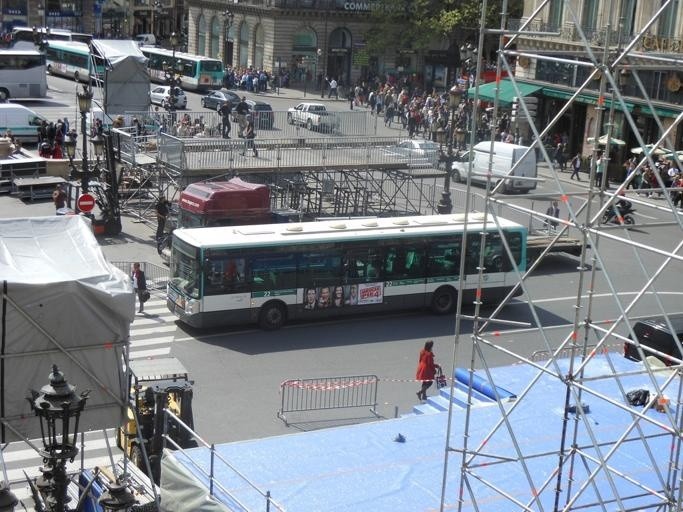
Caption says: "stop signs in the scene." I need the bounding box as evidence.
[76,193,94,212]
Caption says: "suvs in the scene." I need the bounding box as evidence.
[230,101,273,129]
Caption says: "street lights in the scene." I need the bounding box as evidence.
[460,43,481,141]
[154,1,164,42]
[33,3,50,54]
[436,84,462,213]
[317,47,328,98]
[607,68,628,94]
[0,360,136,512]
[219,9,233,66]
[69,84,106,222]
[167,30,184,125]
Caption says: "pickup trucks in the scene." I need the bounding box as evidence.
[287,103,338,133]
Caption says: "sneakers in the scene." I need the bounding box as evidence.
[416,391,428,400]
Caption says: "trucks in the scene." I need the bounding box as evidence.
[160,178,271,262]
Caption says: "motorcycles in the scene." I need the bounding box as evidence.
[602,205,636,229]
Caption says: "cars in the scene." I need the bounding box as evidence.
[200,90,240,112]
[388,139,441,168]
[149,86,186,110]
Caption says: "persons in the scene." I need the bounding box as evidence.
[174,71,181,86]
[224,63,290,92]
[0,22,15,48]
[130,262,146,312]
[224,258,236,281]
[36,104,206,158]
[156,195,171,240]
[349,281,359,305]
[332,284,344,307]
[13,139,22,153]
[89,23,188,52]
[217,100,233,138]
[304,287,318,310]
[240,114,258,156]
[235,96,250,137]
[417,340,441,400]
[4,129,14,144]
[53,184,67,210]
[317,285,330,309]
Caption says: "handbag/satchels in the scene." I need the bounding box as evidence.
[436,375,447,390]
[141,292,150,302]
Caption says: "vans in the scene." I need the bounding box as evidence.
[135,34,155,46]
[622,315,682,366]
[0,103,51,144]
[449,140,537,194]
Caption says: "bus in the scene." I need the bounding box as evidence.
[140,47,224,95]
[8,27,92,60]
[0,50,47,103]
[162,210,528,331]
[40,40,106,86]
